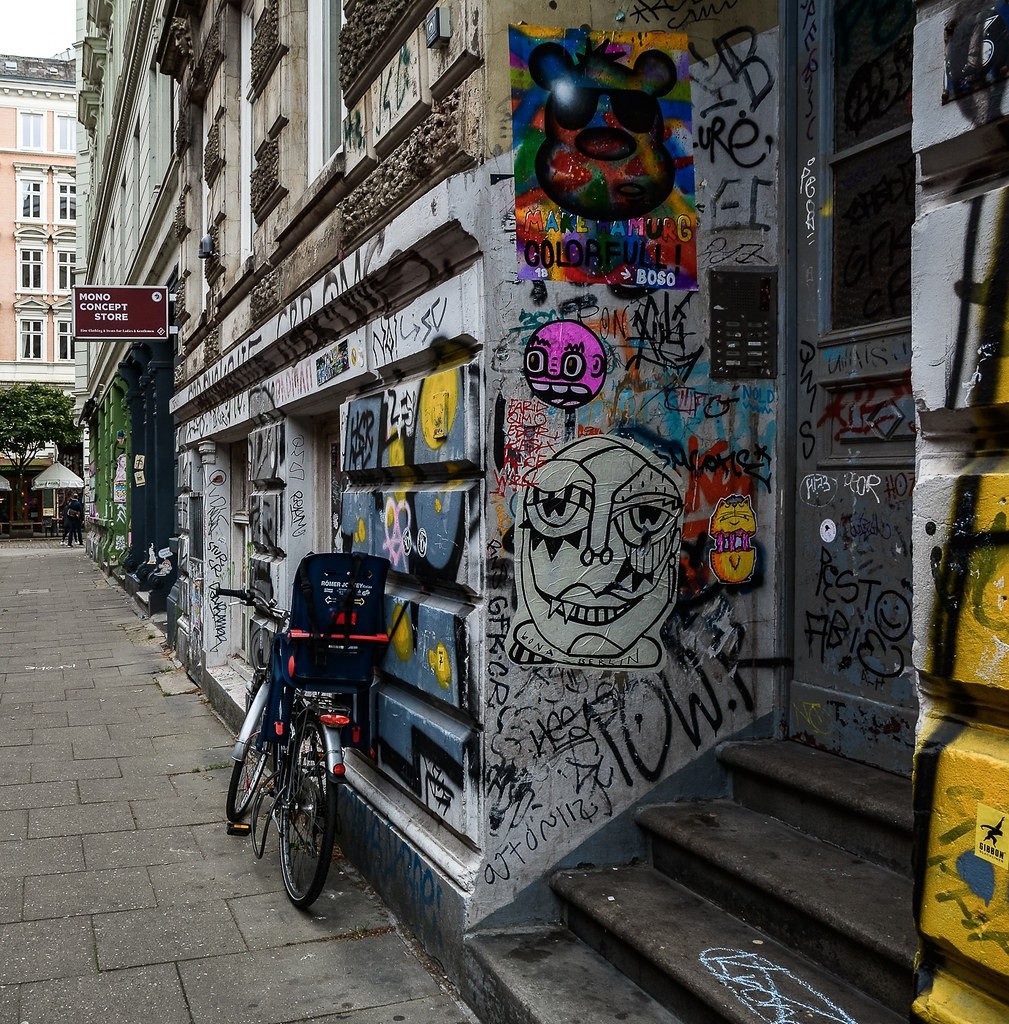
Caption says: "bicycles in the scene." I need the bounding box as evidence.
[216,553,392,910]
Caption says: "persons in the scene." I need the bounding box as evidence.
[60,497,80,546]
[64,493,85,548]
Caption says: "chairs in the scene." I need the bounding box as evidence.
[43,518,56,536]
[276,550,389,691]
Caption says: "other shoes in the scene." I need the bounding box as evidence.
[79,542,83,545]
[61,541,65,544]
[75,541,79,544]
[68,543,73,547]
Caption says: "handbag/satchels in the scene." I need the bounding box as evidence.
[67,508,80,520]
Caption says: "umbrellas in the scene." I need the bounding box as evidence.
[0,475,12,491]
[32,460,85,515]
